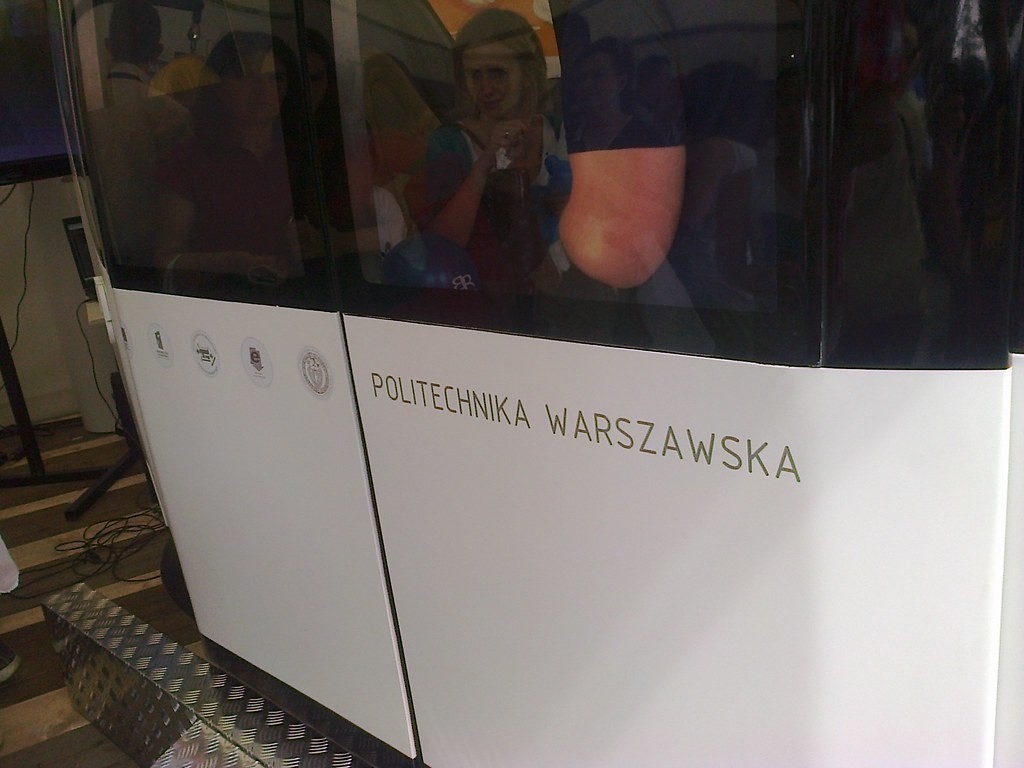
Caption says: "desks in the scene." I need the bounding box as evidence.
[65,295,161,523]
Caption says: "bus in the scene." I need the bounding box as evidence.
[63,2,1024,767]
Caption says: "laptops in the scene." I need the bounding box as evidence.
[63,215,98,302]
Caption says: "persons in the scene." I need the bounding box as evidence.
[415,8,557,293]
[157,31,303,284]
[275,31,408,263]
[829,0,1024,320]
[548,1,807,289]
[81,0,193,261]
[572,43,799,246]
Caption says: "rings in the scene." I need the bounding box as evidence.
[504,128,509,138]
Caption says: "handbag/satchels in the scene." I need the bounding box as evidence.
[404,121,531,304]
